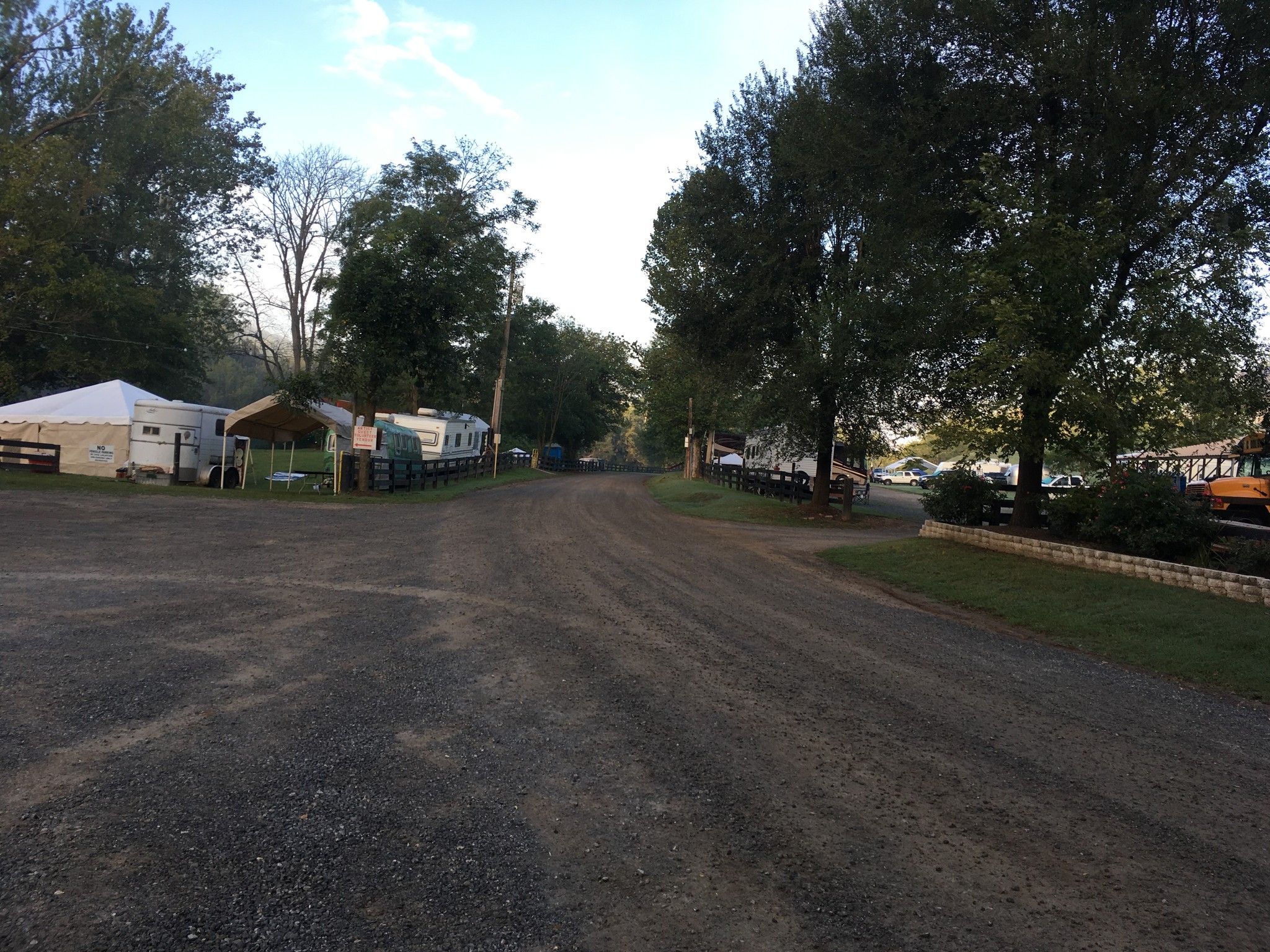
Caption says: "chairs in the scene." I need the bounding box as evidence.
[852,483,870,505]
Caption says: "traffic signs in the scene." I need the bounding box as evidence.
[353,425,377,451]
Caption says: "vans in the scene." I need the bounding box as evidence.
[324,419,423,491]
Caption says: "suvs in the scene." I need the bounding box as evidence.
[879,470,923,486]
[978,472,1007,486]
[871,467,889,483]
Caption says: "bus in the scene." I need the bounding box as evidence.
[1185,432,1270,528]
[829,441,871,500]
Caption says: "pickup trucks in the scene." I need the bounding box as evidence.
[1042,474,1097,501]
[918,469,981,490]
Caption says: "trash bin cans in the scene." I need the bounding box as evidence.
[545,446,562,471]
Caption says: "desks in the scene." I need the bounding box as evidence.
[294,471,334,496]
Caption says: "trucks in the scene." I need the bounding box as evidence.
[336,399,491,477]
[742,426,835,501]
